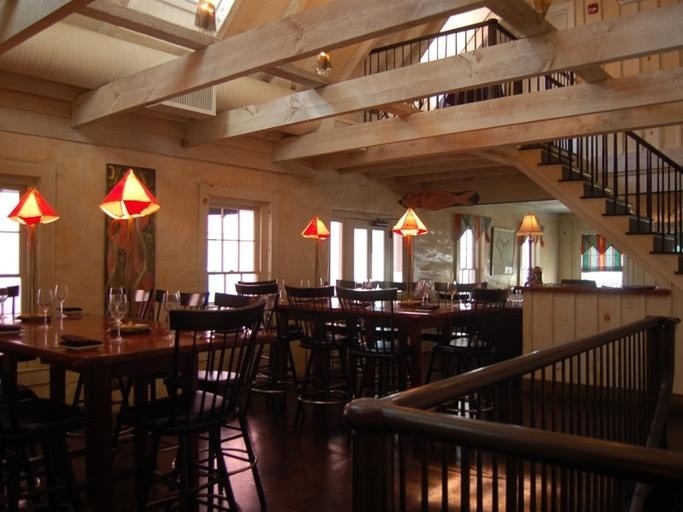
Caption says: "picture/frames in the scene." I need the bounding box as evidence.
[104,163,156,322]
[490,227,516,276]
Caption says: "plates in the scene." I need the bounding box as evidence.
[112,321,151,334]
[16,312,51,322]
[55,307,83,315]
[56,339,105,352]
[0,326,26,336]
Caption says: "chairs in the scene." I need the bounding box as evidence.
[3,337,102,512]
[0,279,522,438]
[116,301,272,509]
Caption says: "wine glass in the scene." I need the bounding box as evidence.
[163,289,183,340]
[272,277,525,307]
[108,287,129,344]
[55,283,68,331]
[37,288,53,329]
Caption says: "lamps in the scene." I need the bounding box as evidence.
[195,0,217,34]
[516,212,545,268]
[316,51,332,78]
[299,216,332,286]
[391,207,429,299]
[7,187,59,321]
[97,169,160,334]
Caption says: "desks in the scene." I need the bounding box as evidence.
[1,313,274,512]
